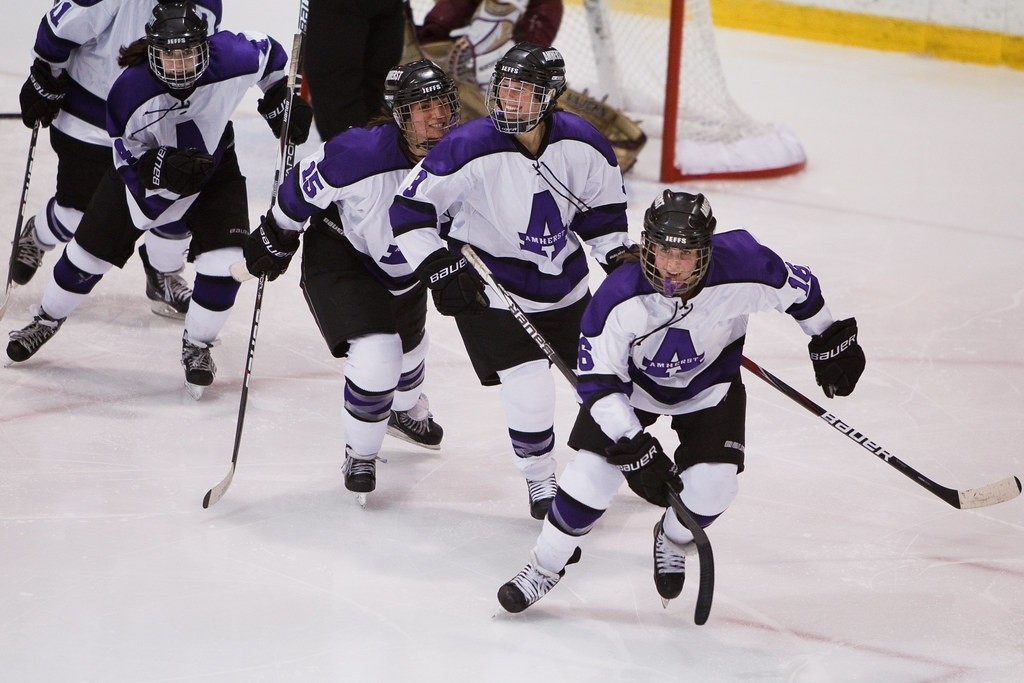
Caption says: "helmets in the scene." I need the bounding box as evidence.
[639,188,717,296]
[483,40,568,135]
[384,57,461,152]
[144,0,210,100]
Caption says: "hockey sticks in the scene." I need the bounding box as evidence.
[203,34,302,510]
[0,0,60,322]
[738,353,1022,509]
[458,242,715,625]
[284,0,309,182]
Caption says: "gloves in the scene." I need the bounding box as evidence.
[257,74,313,145]
[243,208,300,282]
[19,57,71,129]
[605,433,684,508]
[809,317,866,399]
[137,145,215,196]
[413,247,490,317]
[598,246,629,276]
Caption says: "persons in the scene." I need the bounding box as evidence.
[299,0,414,142]
[496,189,866,623]
[418,0,563,126]
[9,0,223,284]
[245,58,460,507]
[5,0,312,403]
[389,41,630,521]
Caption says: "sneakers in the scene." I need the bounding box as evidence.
[8,214,55,285]
[146,264,193,321]
[340,443,387,511]
[4,305,67,367]
[179,327,218,402]
[386,392,443,451]
[496,545,581,614]
[515,431,558,520]
[653,510,686,608]
